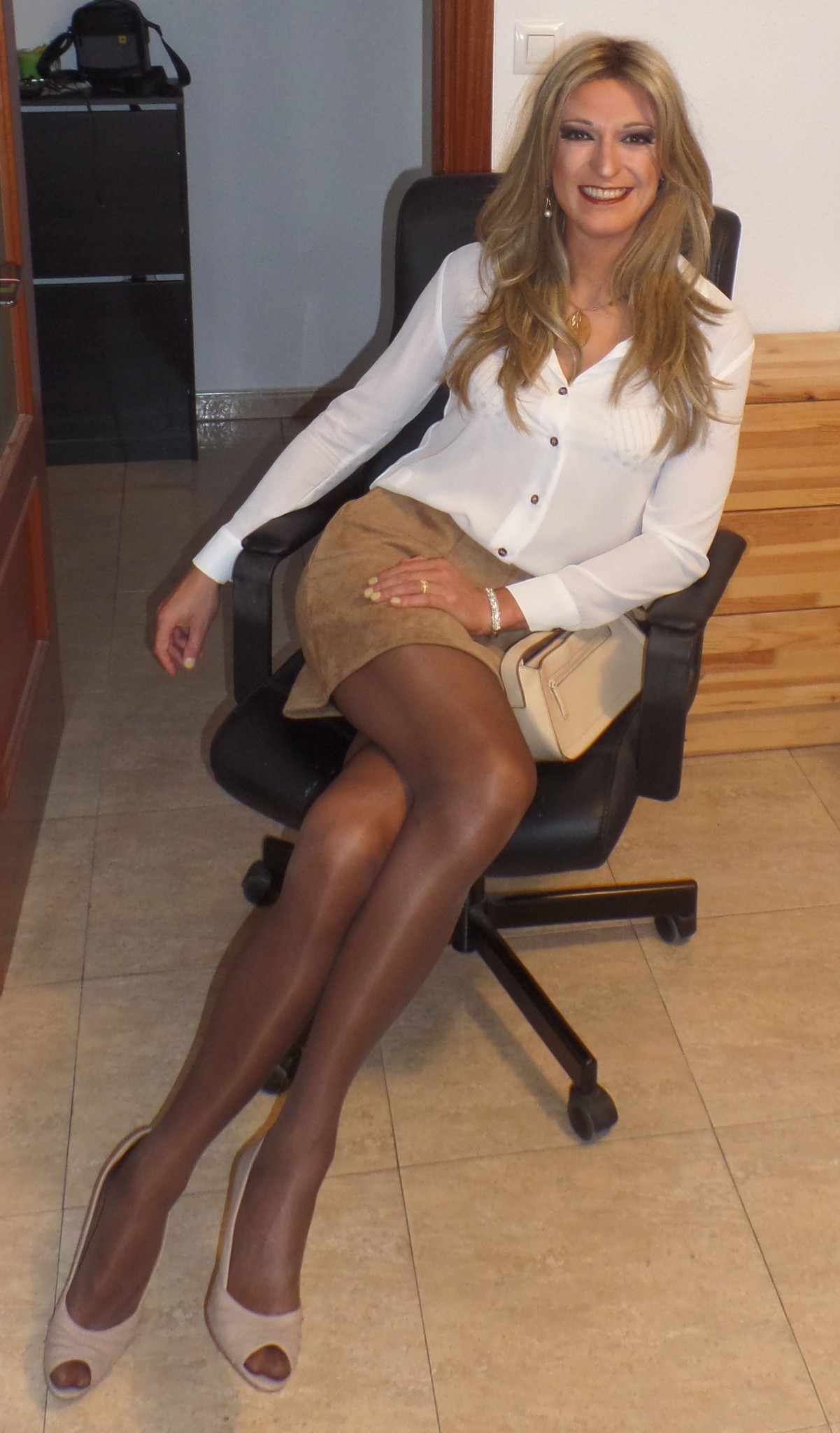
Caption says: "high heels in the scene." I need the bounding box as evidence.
[42,1126,170,1402]
[202,1135,305,1390]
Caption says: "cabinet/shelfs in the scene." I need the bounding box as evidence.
[19,77,199,463]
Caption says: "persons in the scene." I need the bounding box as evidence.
[43,30,754,1401]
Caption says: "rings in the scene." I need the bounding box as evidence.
[420,580,429,594]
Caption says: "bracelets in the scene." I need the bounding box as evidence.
[482,587,500,640]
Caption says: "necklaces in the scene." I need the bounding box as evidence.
[566,295,626,349]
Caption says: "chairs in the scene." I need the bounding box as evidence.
[208,171,750,1136]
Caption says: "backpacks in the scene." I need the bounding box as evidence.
[36,0,191,92]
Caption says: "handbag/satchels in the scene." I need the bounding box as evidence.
[501,612,647,763]
[16,41,61,79]
